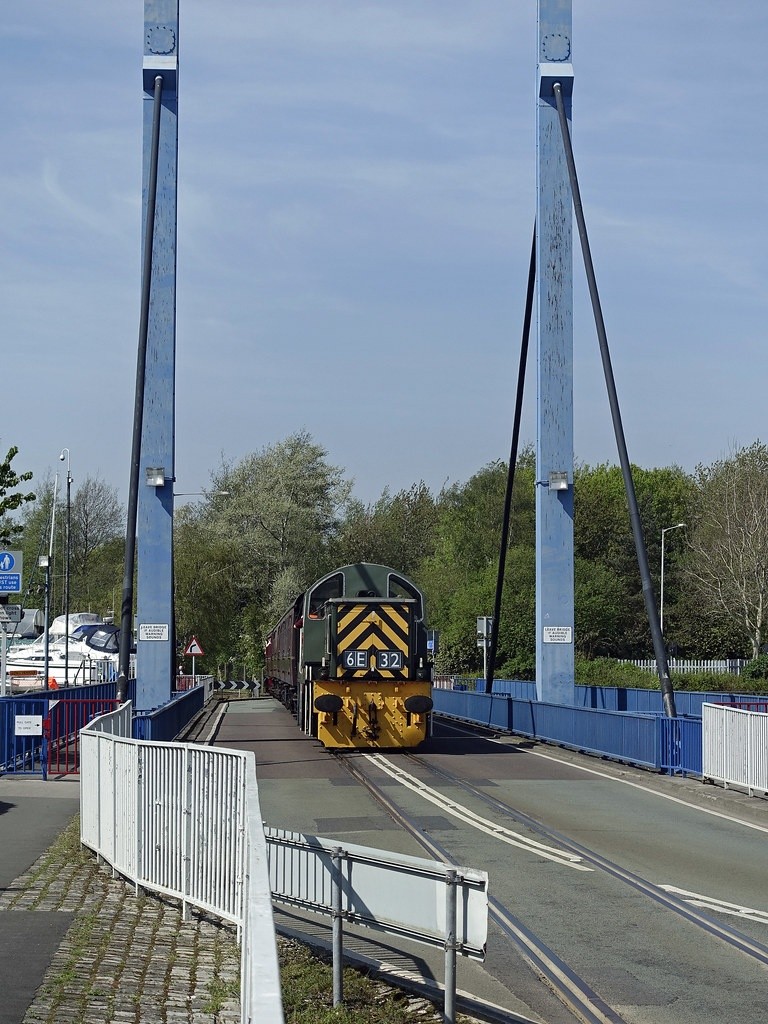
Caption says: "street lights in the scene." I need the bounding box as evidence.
[59,446,75,689]
[660,522,687,639]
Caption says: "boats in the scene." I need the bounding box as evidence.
[6,645,102,692]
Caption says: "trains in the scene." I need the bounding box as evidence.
[264,559,441,753]
